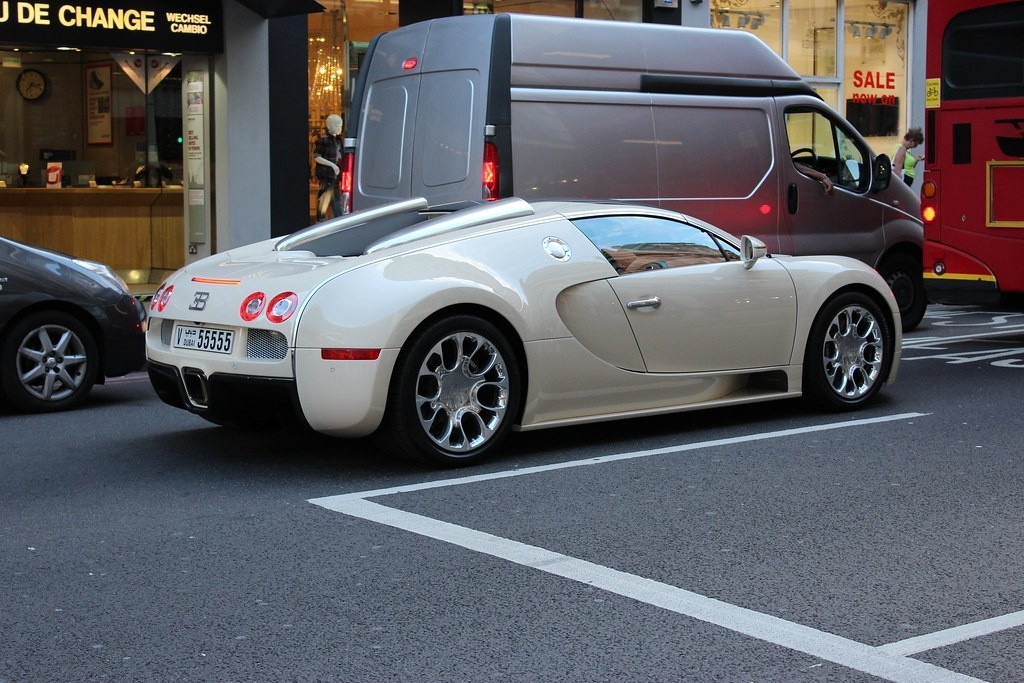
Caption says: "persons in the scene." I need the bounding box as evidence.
[890,128,925,187]
[794,163,834,198]
[313,114,344,223]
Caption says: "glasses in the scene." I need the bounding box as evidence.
[913,140,918,147]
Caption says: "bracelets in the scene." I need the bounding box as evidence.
[821,174,826,181]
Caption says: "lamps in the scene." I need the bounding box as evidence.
[879,24,892,38]
[852,24,862,39]
[865,24,876,38]
[738,14,749,27]
[750,14,765,30]
[722,14,730,25]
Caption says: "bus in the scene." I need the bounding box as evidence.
[921,1,1024,299]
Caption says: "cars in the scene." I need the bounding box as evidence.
[0,235,148,415]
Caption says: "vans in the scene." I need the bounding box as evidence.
[338,12,929,333]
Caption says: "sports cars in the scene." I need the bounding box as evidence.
[146,196,904,470]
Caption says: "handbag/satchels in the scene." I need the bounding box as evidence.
[890,146,905,181]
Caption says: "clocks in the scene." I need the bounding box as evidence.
[16,69,46,100]
